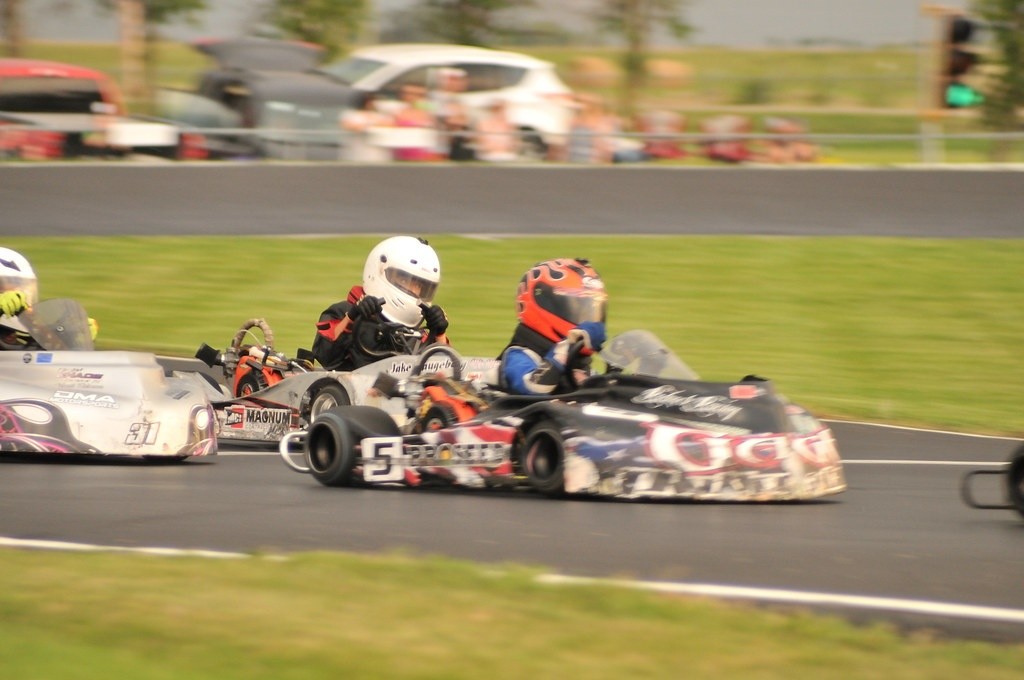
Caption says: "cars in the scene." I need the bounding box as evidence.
[189,35,361,160]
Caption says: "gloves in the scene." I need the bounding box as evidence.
[420,304,449,337]
[543,321,607,373]
[71,316,98,345]
[347,294,384,322]
[0,290,29,317]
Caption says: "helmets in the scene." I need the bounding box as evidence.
[362,236,441,328]
[516,257,608,356]
[0,246,38,333]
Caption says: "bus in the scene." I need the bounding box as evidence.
[0,58,128,160]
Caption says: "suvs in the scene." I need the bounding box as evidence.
[300,43,574,161]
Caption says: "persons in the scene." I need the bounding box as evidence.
[472,99,519,161]
[434,68,470,161]
[498,258,624,401]
[311,232,450,374]
[387,84,441,161]
[563,95,615,163]
[339,90,391,162]
[1,247,98,350]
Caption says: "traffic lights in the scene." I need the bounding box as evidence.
[943,12,987,107]
